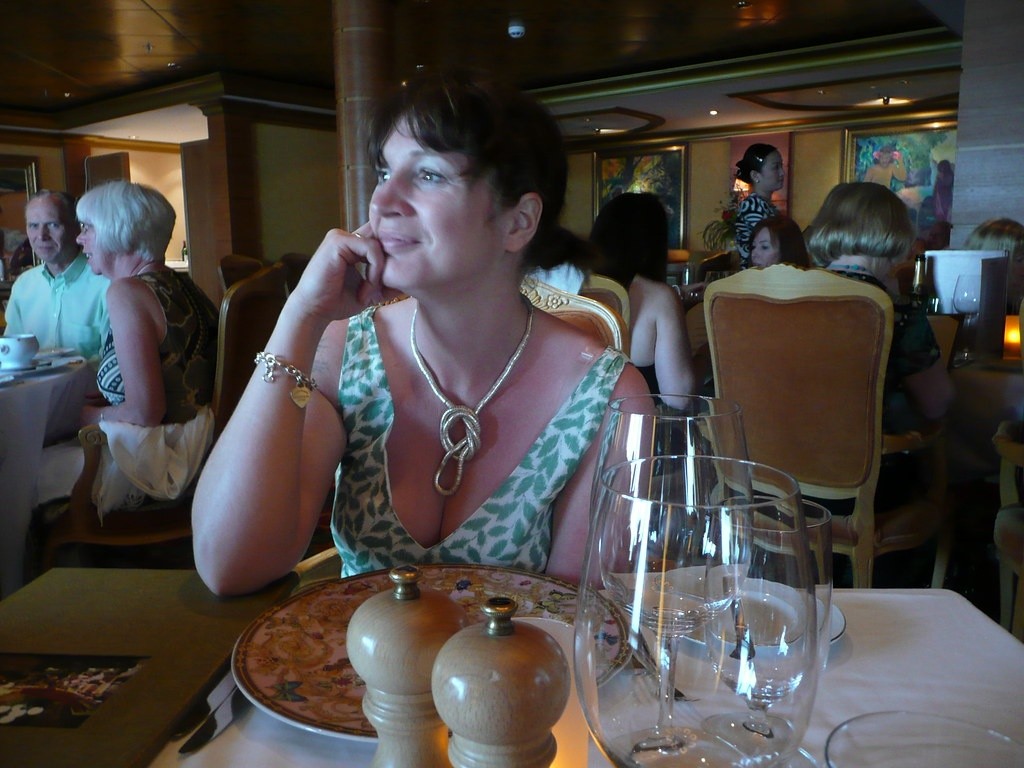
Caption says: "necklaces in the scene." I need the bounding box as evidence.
[410,291,534,497]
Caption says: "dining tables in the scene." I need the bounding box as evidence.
[1,568,1022,767]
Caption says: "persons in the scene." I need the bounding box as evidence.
[22,177,220,584]
[3,189,112,372]
[750,213,810,271]
[191,67,659,599]
[735,143,786,270]
[962,218,1024,313]
[800,181,1000,624]
[580,192,708,473]
[927,221,950,250]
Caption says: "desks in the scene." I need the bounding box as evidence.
[0,350,89,592]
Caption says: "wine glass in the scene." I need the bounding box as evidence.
[953,274,982,364]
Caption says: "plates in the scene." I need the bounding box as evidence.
[824,710,1024,768]
[230,563,633,745]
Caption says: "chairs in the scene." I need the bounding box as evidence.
[991,418,1024,643]
[697,265,957,591]
[32,254,631,575]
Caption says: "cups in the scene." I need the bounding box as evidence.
[570,392,834,768]
[0,333,39,372]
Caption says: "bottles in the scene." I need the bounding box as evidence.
[912,253,928,314]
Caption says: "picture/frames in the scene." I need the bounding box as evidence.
[839,117,959,236]
[0,153,38,201]
[591,140,688,252]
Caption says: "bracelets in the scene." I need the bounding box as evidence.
[101,407,110,421]
[254,352,319,408]
